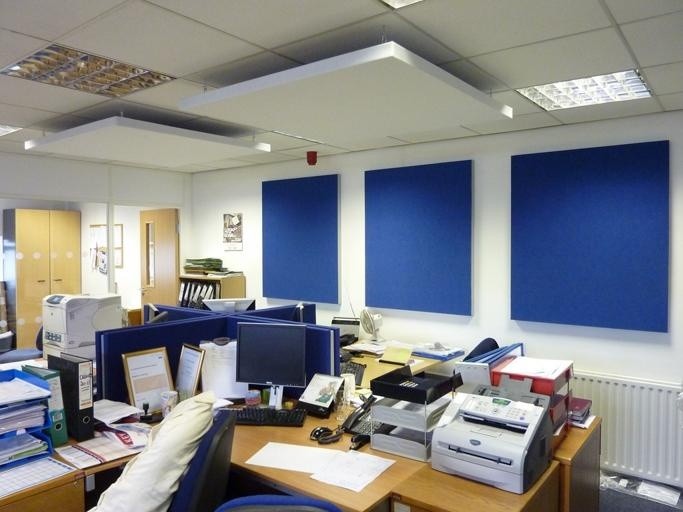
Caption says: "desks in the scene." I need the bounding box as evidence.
[0,386,430,512]
[335,336,464,389]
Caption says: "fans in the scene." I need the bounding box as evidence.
[358,309,385,344]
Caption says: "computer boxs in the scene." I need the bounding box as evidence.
[42,293,121,361]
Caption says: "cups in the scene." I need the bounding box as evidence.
[244,390,261,405]
[161,391,177,418]
[329,381,351,425]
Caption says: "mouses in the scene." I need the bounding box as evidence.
[309,426,332,440]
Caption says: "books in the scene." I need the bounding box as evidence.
[0,352,97,466]
[183,258,230,277]
[460,342,592,428]
[178,280,220,308]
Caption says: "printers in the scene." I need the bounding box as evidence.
[430,373,554,494]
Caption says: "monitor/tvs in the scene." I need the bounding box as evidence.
[144,310,168,324]
[235,322,307,410]
[147,303,159,321]
[291,302,305,323]
[200,298,255,313]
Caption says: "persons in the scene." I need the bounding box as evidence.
[317,381,338,403]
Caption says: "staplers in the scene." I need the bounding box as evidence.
[348,433,370,451]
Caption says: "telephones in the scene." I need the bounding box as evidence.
[341,395,397,440]
[338,334,358,347]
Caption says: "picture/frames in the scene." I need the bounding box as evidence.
[175,341,205,401]
[120,344,174,409]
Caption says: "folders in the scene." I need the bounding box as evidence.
[21,352,94,448]
[177,281,221,310]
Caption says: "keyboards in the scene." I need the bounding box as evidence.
[341,361,366,386]
[234,409,305,426]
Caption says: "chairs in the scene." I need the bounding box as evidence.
[213,495,344,511]
[0,325,45,363]
[164,394,236,512]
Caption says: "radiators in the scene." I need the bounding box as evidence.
[553,367,683,490]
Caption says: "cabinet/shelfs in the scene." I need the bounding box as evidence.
[175,270,246,311]
[551,415,602,511]
[387,448,562,511]
[1,209,82,349]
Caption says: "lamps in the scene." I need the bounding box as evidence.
[170,26,514,156]
[13,109,272,176]
